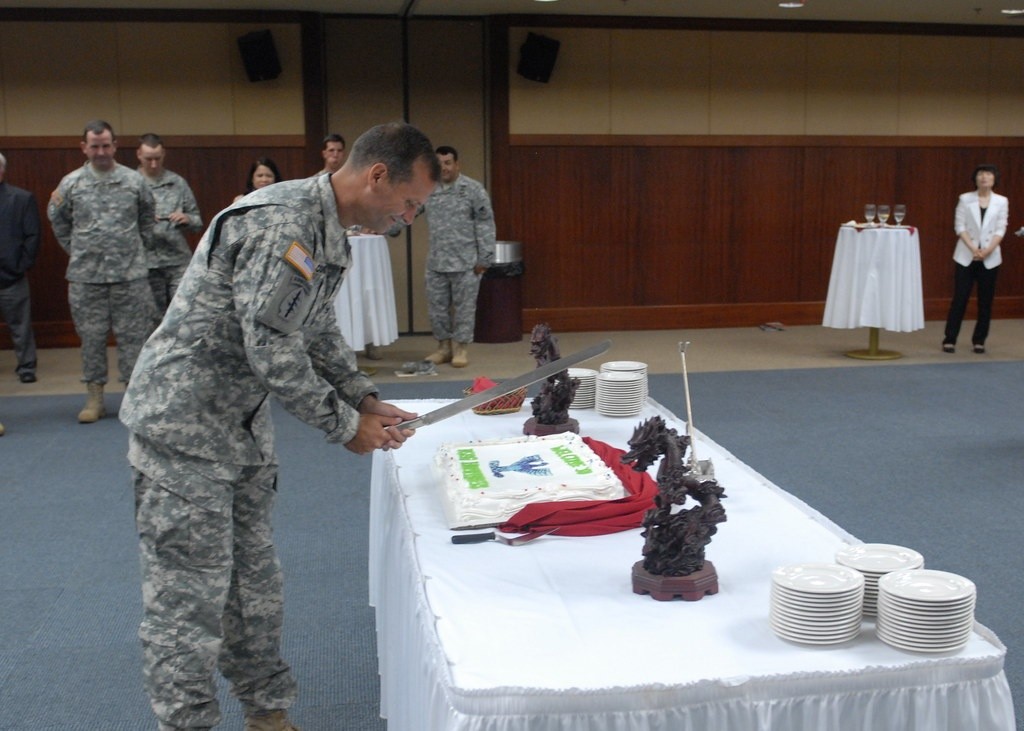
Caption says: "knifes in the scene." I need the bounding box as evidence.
[382,338,611,433]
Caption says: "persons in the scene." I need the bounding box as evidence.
[388,146,496,367]
[234,157,281,201]
[137,132,203,315]
[47,120,159,422]
[0,152,41,382]
[118,125,441,731]
[941,164,1009,352]
[315,133,347,173]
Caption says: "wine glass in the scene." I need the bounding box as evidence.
[878,205,890,229]
[893,204,906,228]
[864,203,876,222]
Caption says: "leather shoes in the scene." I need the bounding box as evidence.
[943,344,954,352]
[20,373,36,383]
[975,344,984,353]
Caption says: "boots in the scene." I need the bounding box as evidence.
[424,339,452,364]
[451,342,469,368]
[77,383,106,423]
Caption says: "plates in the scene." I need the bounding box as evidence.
[768,562,864,645]
[835,542,924,617]
[566,361,649,417]
[876,569,977,653]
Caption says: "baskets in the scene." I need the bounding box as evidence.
[462,383,528,414]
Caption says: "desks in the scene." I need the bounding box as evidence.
[330,231,398,377]
[822,224,925,359]
[368,398,1016,731]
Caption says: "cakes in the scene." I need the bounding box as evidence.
[432,431,626,529]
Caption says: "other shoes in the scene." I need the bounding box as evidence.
[365,344,386,359]
[244,710,299,731]
[0,424,5,436]
